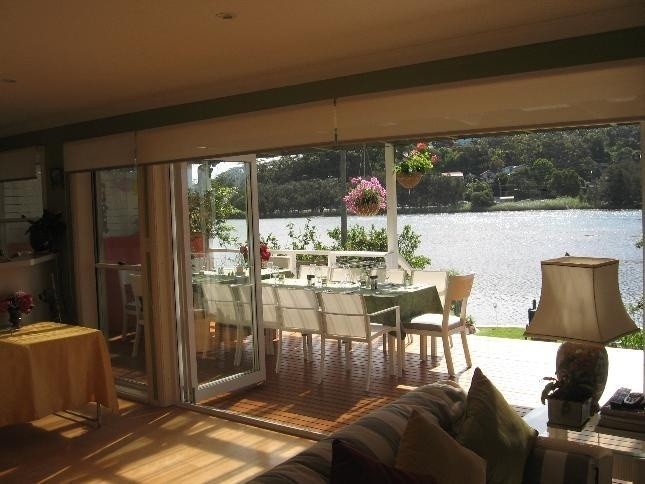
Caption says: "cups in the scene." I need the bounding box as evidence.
[279,274,285,281]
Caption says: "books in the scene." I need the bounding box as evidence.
[596,386,645,437]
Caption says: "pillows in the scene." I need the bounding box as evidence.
[331,365,540,483]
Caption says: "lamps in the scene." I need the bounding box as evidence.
[523,256,642,401]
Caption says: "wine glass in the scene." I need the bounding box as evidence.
[268,262,279,284]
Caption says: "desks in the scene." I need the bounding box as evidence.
[0,322,103,427]
[522,404,645,484]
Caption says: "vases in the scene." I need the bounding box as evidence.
[9,313,21,328]
[396,171,424,188]
[357,202,380,214]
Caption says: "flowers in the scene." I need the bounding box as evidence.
[394,139,439,174]
[342,176,387,214]
[1,291,35,315]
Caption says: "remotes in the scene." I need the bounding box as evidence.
[624,392,643,408]
[609,387,631,406]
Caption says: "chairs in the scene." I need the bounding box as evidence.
[191,255,475,390]
[117,263,144,357]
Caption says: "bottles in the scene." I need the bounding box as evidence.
[307,274,315,287]
[370,275,378,289]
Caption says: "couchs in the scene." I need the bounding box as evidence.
[243,381,612,484]
